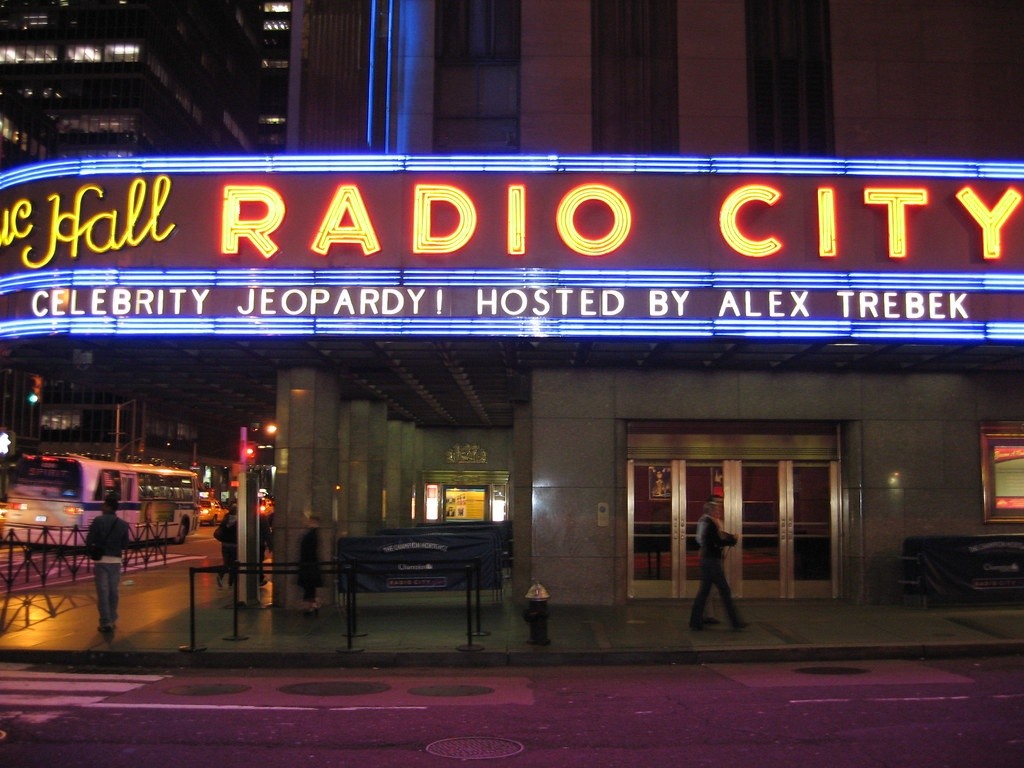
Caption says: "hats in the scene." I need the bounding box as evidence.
[230,503,237,512]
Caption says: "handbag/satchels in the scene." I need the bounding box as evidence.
[87,542,102,561]
[213,526,223,541]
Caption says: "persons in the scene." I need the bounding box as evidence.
[259,504,272,585]
[87,497,129,631]
[297,517,324,612]
[689,502,750,628]
[214,504,237,589]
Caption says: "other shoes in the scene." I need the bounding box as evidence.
[98,623,116,632]
[692,624,709,630]
[259,580,268,587]
[704,616,720,625]
[216,576,223,587]
[730,621,747,629]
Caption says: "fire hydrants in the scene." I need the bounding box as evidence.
[522,581,553,646]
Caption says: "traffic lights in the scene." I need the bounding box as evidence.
[0,431,16,457]
[138,439,145,453]
[246,441,255,459]
[26,374,42,405]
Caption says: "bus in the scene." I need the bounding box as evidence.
[0,451,201,555]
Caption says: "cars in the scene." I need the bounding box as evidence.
[259,498,274,517]
[200,498,229,526]
